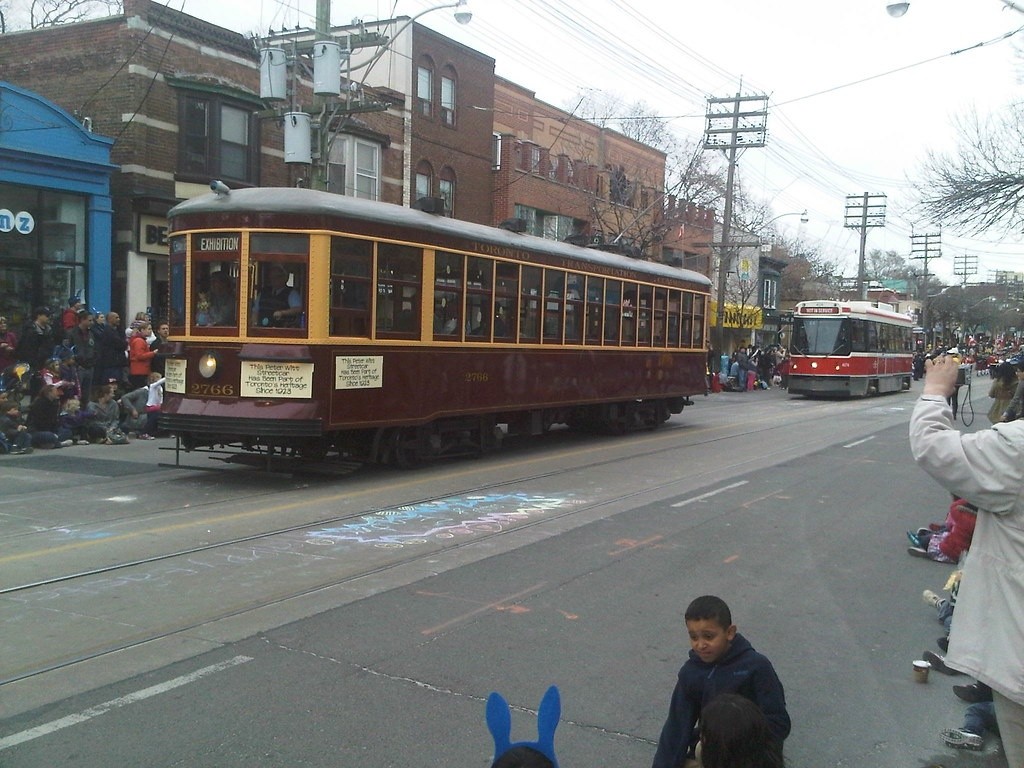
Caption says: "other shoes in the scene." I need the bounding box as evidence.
[953,685,992,703]
[922,650,956,675]
[923,590,939,607]
[938,729,983,751]
[57,439,74,446]
[906,523,944,558]
[11,446,33,455]
[137,433,154,441]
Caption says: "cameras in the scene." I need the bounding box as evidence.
[955,365,971,386]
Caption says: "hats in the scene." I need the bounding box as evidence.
[69,296,80,307]
[35,306,54,315]
[105,378,119,385]
[946,347,959,354]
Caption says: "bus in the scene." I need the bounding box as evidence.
[158,179,713,471]
[788,299,913,400]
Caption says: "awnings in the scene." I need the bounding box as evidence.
[710,300,763,329]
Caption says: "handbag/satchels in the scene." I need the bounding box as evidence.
[106,427,129,445]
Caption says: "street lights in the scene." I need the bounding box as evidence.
[923,283,966,350]
[962,295,997,346]
[715,209,808,390]
[309,0,472,189]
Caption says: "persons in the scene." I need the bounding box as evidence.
[652,596,791,767]
[485,747,561,768]
[907,332,1024,768]
[0,296,170,454]
[194,272,237,326]
[394,298,504,336]
[707,342,790,393]
[251,263,303,327]
